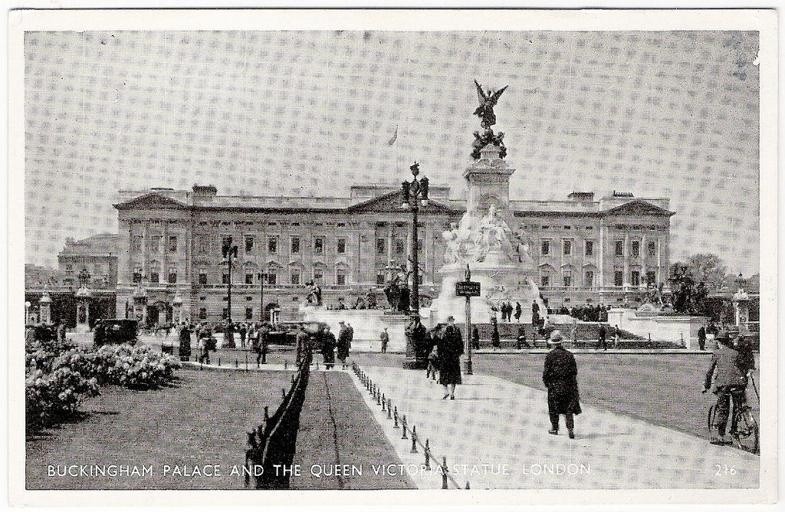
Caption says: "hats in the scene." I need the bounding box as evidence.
[714,331,733,341]
[547,330,566,344]
[729,325,740,334]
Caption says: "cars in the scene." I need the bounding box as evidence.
[248,321,336,354]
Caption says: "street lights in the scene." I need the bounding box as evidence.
[258,268,267,325]
[222,235,238,349]
[25,301,31,338]
[400,160,430,369]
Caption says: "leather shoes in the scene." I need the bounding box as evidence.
[569,429,574,438]
[549,429,558,434]
[710,439,724,446]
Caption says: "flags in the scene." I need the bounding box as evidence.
[389,130,397,145]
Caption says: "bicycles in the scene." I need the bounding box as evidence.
[700,378,760,455]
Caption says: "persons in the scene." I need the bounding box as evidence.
[336,265,414,314]
[542,331,581,439]
[470,91,507,160]
[489,299,628,350]
[703,333,746,446]
[134,316,464,400]
[698,327,706,350]
[442,205,533,281]
[305,279,322,307]
[706,320,736,345]
[728,327,756,404]
[641,263,707,313]
[471,324,480,349]
